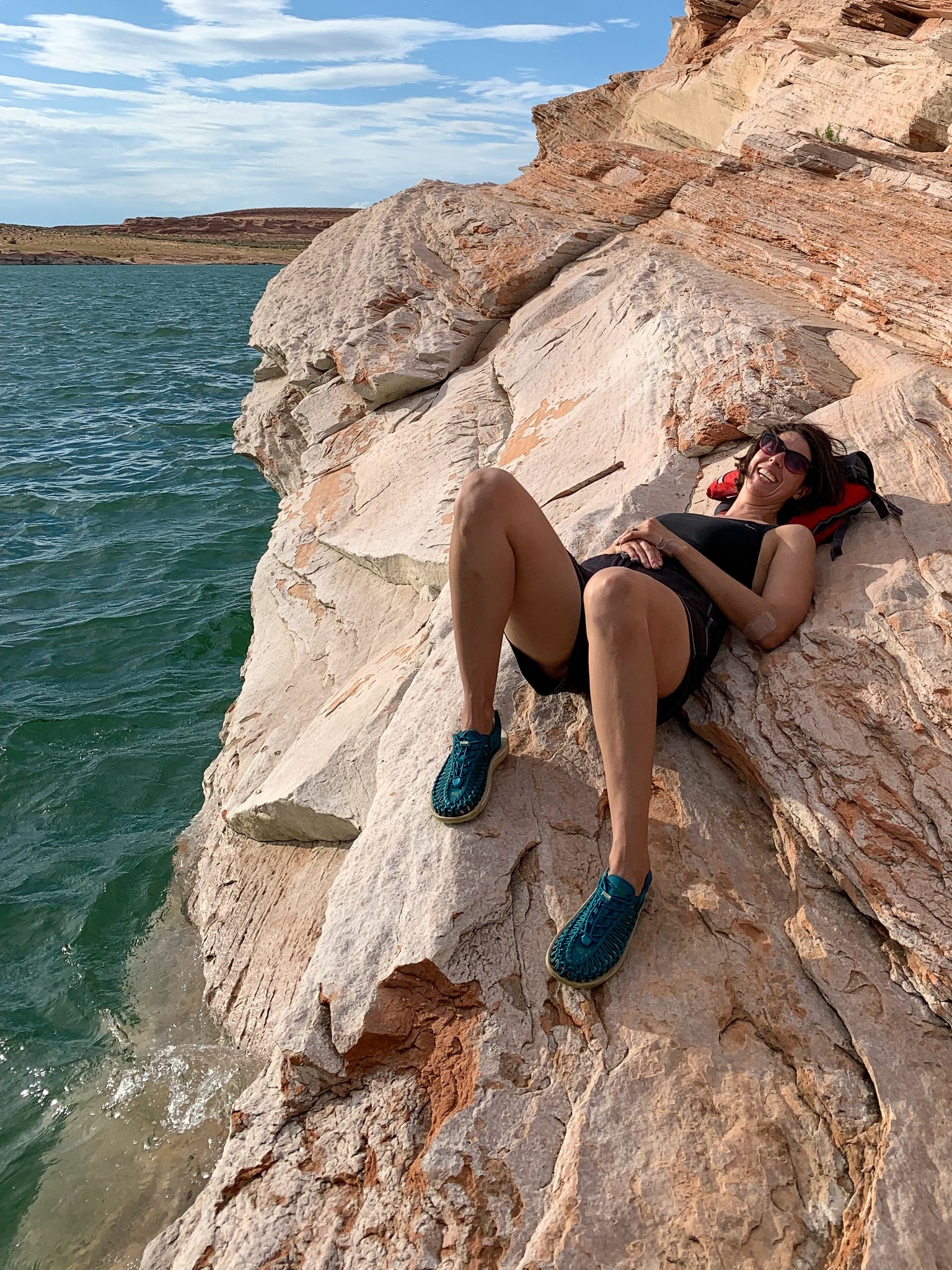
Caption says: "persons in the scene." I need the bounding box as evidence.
[429,423,846,989]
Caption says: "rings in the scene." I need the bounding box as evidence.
[631,526,637,531]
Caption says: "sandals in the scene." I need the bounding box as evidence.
[430,710,509,823]
[545,865,653,988]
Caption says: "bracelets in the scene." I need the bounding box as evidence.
[615,544,622,554]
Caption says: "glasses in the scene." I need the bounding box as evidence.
[759,431,813,475]
